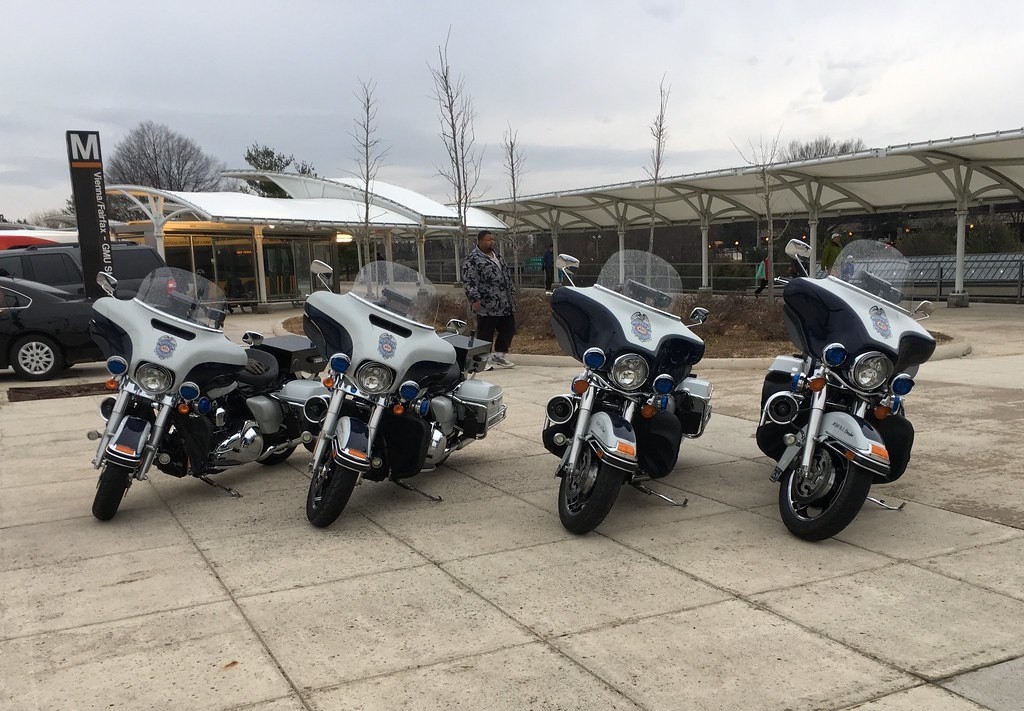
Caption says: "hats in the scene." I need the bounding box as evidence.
[832,233,841,239]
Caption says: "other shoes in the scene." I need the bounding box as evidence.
[754,291,758,298]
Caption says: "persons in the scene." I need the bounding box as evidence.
[225,273,245,315]
[463,230,518,371]
[820,233,844,274]
[541,244,553,292]
[754,255,769,298]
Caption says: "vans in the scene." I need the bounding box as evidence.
[0,241,176,306]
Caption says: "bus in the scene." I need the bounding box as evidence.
[109,220,301,307]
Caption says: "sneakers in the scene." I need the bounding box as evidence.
[488,354,515,368]
[483,363,494,371]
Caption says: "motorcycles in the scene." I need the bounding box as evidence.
[541,250,714,535]
[87,266,328,522]
[277,259,508,529]
[755,238,937,543]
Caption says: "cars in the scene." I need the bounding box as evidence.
[0,276,106,382]
